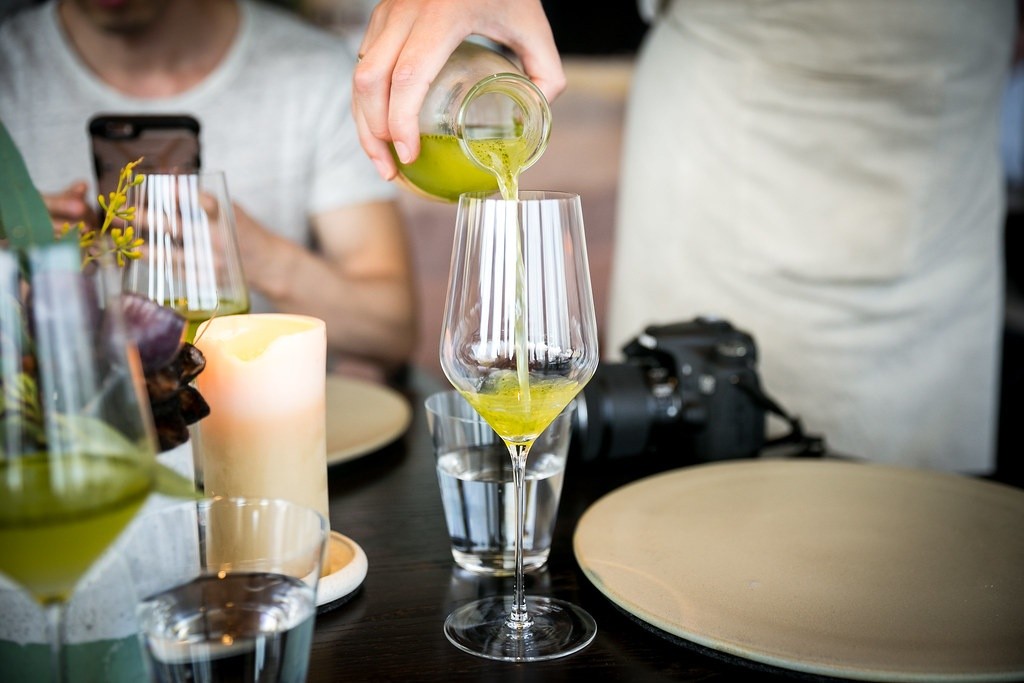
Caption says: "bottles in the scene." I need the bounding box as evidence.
[388,41,554,199]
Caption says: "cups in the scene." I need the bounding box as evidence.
[122,170,252,346]
[425,390,578,576]
[113,499,328,683]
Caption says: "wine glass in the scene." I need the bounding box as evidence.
[0,239,159,683]
[439,190,600,662]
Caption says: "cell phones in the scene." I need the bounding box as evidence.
[90,115,200,223]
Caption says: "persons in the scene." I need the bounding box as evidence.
[0,0,420,391]
[351,0,1020,480]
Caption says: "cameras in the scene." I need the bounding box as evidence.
[557,318,766,514]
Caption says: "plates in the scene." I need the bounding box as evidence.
[188,377,411,482]
[573,457,1024,683]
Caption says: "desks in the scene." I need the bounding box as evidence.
[0,361,1024,683]
